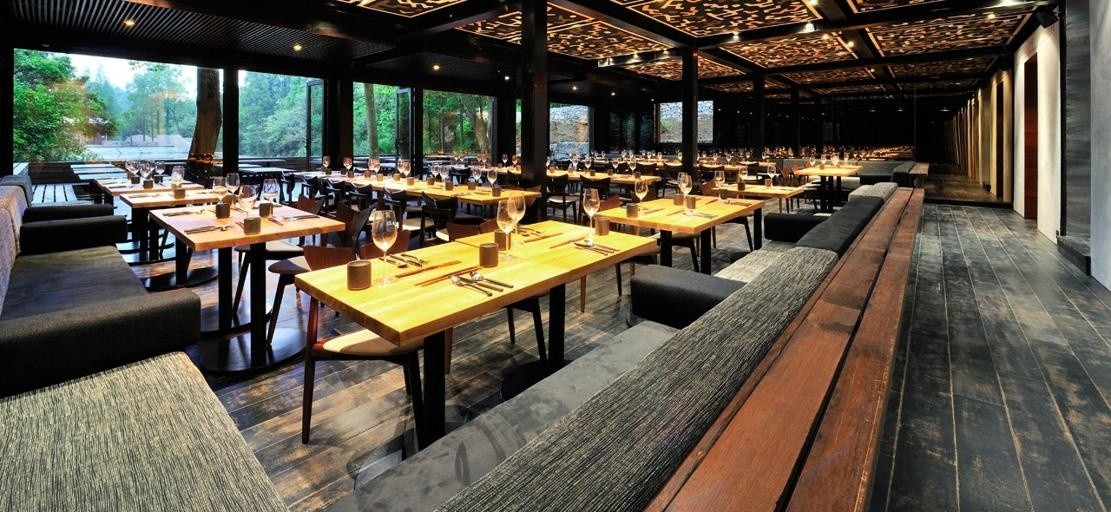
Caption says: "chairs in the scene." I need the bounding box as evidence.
[69,149,547,374]
[482,136,916,237]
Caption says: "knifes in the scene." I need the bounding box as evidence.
[458,273,503,293]
[378,253,426,272]
[727,200,752,207]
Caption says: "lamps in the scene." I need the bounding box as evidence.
[598,16,819,71]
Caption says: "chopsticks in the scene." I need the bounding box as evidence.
[416,266,479,288]
[395,259,462,279]
[185,212,320,243]
[522,232,563,245]
[549,234,588,249]
[643,197,717,217]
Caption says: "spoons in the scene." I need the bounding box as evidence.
[683,210,717,220]
[447,273,494,298]
[512,223,541,237]
[466,269,516,289]
[572,239,620,256]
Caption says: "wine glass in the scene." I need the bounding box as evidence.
[372,209,399,286]
[502,143,755,181]
[508,191,527,247]
[143,159,282,220]
[767,164,777,178]
[678,172,694,195]
[757,146,905,170]
[582,189,601,242]
[321,150,502,199]
[713,171,726,205]
[634,177,650,210]
[739,165,748,182]
[497,199,518,262]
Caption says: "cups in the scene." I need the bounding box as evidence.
[479,243,499,269]
[625,202,639,218]
[688,196,697,209]
[672,193,684,206]
[736,182,746,191]
[494,228,512,251]
[346,259,372,290]
[595,214,612,236]
[766,178,772,188]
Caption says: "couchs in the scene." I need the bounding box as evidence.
[0,159,294,512]
[306,175,901,512]
